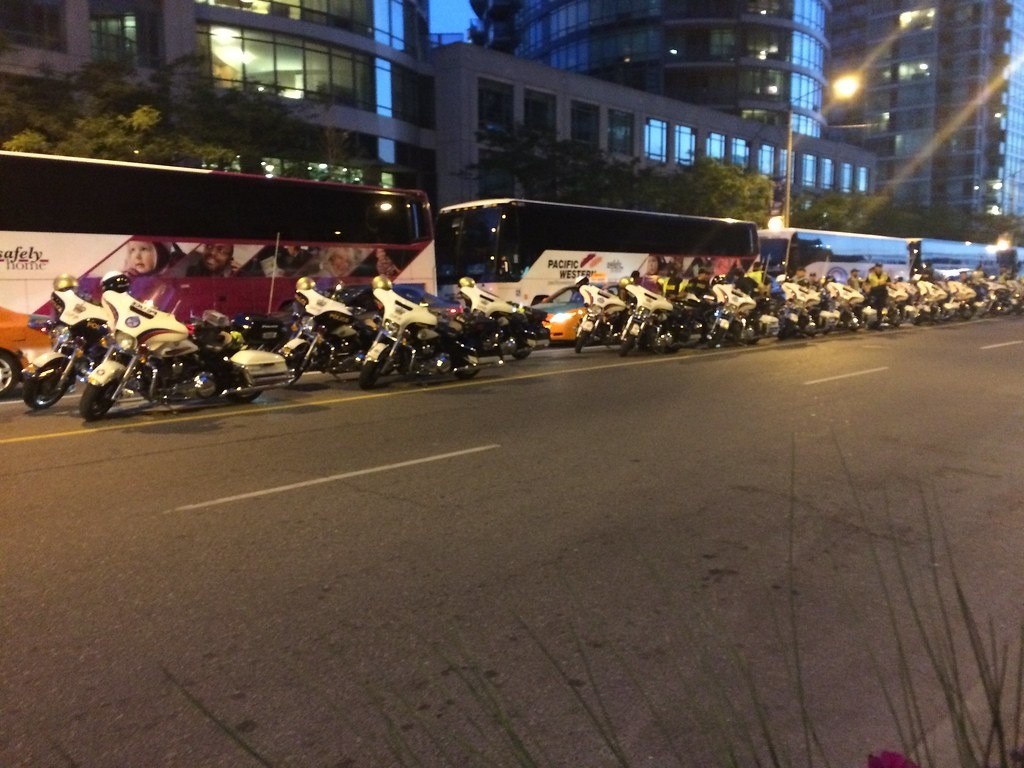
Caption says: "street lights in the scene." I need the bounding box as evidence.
[784,73,860,228]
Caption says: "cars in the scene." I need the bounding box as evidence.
[529,280,628,341]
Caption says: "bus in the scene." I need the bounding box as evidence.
[0,150,437,400]
[437,198,762,312]
[756,227,1024,287]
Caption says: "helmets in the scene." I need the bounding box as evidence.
[458,277,475,286]
[53,275,80,292]
[618,277,631,288]
[296,277,317,291]
[574,276,589,288]
[372,276,393,291]
[99,270,131,292]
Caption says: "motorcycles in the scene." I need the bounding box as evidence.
[22,270,290,424]
[279,275,552,391]
[573,270,1024,359]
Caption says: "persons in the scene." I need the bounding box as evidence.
[646,255,668,276]
[733,262,772,298]
[848,261,893,331]
[124,239,168,280]
[186,242,241,279]
[250,244,313,277]
[685,269,712,297]
[914,258,934,282]
[794,267,809,287]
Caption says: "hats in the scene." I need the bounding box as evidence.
[875,262,883,267]
